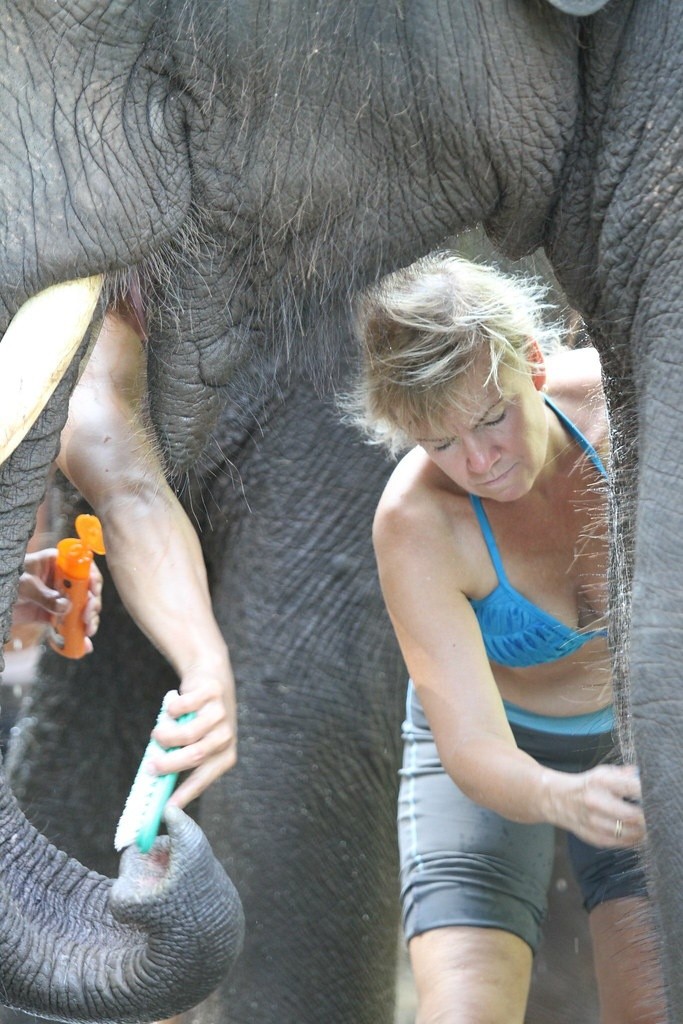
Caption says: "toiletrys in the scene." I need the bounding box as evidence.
[46,513,107,659]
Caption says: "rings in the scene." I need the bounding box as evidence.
[614,819,623,838]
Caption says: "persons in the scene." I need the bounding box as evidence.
[330,250,669,1024]
[1,284,237,813]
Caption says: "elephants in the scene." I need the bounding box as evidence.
[2,3,683,1024]
